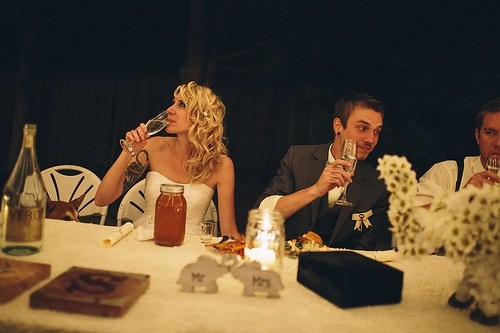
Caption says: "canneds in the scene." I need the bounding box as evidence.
[154,184,187,247]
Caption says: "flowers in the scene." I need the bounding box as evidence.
[378,154,500,304]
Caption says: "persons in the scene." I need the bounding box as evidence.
[412,101,500,256]
[95,81,241,240]
[253,94,393,251]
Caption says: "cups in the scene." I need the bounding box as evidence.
[200,222,213,243]
[485,157,500,186]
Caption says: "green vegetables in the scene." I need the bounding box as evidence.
[285,239,302,259]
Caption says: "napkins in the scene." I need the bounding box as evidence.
[103,222,134,247]
[350,247,397,263]
[136,225,196,243]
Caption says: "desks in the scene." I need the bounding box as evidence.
[0,218,500,333]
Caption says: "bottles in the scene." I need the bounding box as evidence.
[244,209,285,282]
[154,185,186,246]
[0,124,47,256]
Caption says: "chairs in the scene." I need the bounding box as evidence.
[19,165,107,224]
[115,179,218,238]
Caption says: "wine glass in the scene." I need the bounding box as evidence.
[120,111,172,156]
[334,139,357,206]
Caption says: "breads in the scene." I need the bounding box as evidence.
[303,232,323,247]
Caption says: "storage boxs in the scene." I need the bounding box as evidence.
[296,251,404,309]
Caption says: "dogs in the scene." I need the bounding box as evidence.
[46,191,86,223]
